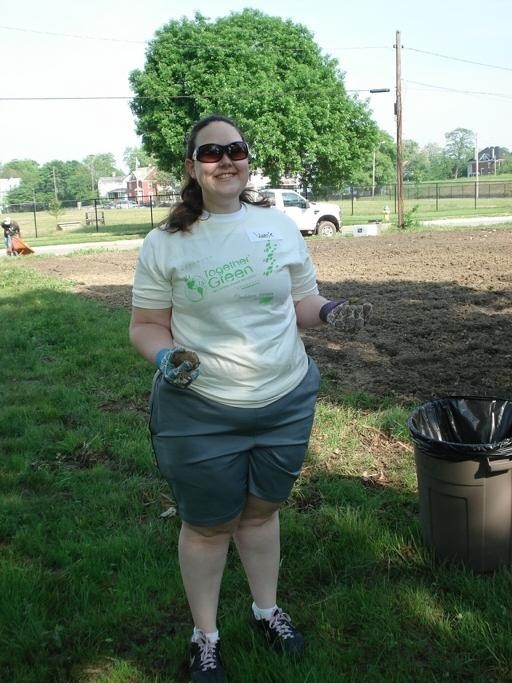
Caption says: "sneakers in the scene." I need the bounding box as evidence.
[252,601,305,662]
[190,630,228,683]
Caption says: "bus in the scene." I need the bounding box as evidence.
[349,186,372,195]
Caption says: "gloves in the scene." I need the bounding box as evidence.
[154,347,201,389]
[320,300,373,334]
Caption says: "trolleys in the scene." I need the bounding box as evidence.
[6,232,35,259]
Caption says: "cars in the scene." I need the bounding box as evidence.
[340,189,358,199]
[113,201,139,208]
[324,190,342,200]
[94,200,120,208]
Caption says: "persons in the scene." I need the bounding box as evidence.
[1,216,22,255]
[125,113,372,682]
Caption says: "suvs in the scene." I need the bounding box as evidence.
[242,188,343,239]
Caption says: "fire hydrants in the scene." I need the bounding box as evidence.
[380,206,390,223]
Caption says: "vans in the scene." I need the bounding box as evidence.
[2,202,50,212]
[55,200,89,209]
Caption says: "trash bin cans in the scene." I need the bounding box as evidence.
[409,395,512,574]
[367,219,382,236]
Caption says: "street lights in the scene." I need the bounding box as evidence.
[372,141,387,201]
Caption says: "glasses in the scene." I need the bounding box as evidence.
[192,141,249,163]
